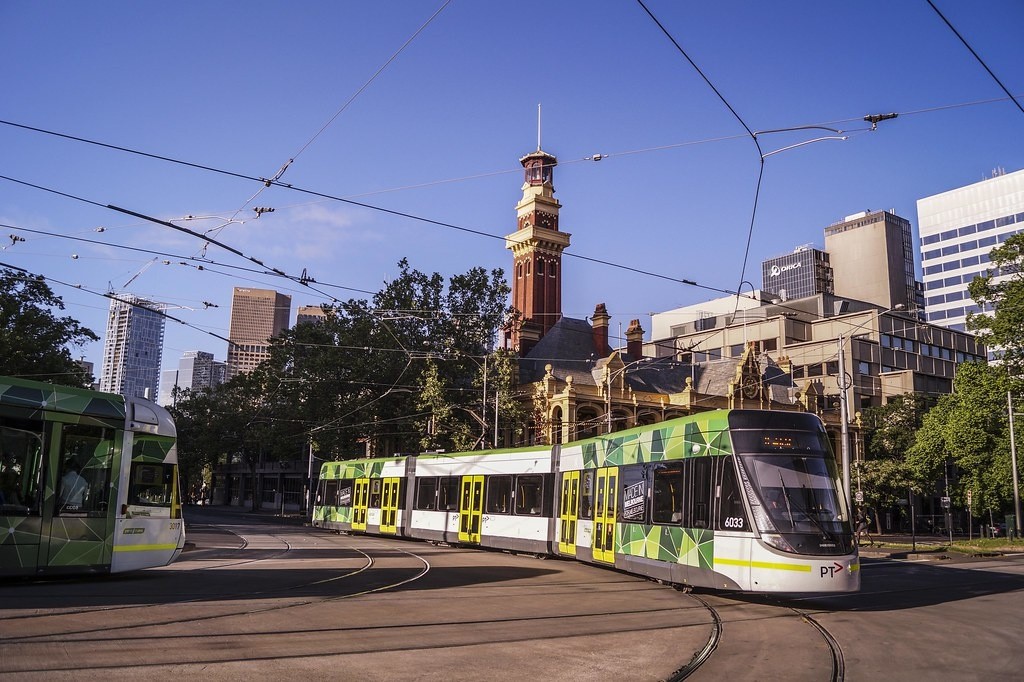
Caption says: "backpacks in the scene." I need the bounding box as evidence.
[865,514,873,524]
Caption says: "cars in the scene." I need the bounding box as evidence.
[989,523,1007,538]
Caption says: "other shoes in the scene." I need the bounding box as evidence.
[857,540,860,545]
[871,540,873,545]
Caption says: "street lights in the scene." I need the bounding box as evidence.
[839,304,908,529]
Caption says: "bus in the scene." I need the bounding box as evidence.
[311,410,861,603]
[0,376,188,580]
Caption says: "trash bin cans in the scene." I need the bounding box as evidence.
[1005,515,1016,536]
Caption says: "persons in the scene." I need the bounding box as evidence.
[765,488,790,512]
[60,449,104,508]
[0,453,25,506]
[856,507,873,547]
